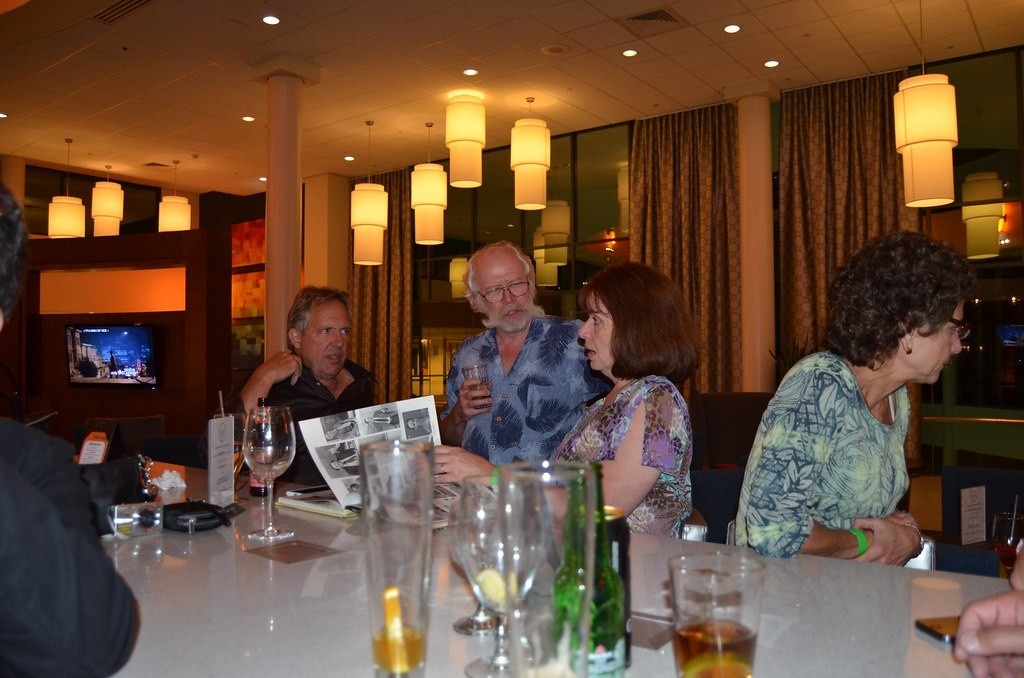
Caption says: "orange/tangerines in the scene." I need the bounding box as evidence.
[384,586,411,674]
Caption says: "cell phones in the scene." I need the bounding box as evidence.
[914,614,961,652]
[287,485,330,496]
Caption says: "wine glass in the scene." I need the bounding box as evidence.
[458,473,555,678]
[446,502,507,635]
[213,414,250,504]
[243,406,296,540]
[992,513,1024,579]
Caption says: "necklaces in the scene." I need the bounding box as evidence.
[606,378,634,402]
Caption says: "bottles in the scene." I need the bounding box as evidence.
[552,462,627,678]
[248,397,277,496]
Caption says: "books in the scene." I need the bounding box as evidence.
[276,393,502,532]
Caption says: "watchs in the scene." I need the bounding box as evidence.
[903,523,924,557]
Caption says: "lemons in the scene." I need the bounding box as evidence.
[476,569,507,605]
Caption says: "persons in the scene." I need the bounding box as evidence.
[438,235,617,490]
[324,417,358,442]
[108,351,119,378]
[949,538,1024,678]
[328,441,362,476]
[0,181,141,678]
[405,413,431,434]
[363,406,399,432]
[348,480,363,494]
[410,258,694,540]
[733,232,973,569]
[231,283,375,488]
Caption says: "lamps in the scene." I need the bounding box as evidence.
[892,0,957,208]
[962,169,1004,258]
[350,122,389,265]
[48,139,85,239]
[509,97,550,210]
[445,94,486,188]
[449,161,634,301]
[92,165,124,236]
[159,159,191,231]
[411,122,448,246]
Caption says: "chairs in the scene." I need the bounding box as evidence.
[910,413,1024,585]
[687,391,775,547]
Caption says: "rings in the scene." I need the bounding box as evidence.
[441,463,443,471]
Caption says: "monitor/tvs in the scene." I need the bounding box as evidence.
[64,323,161,389]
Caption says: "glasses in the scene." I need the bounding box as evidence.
[949,316,971,340]
[476,278,531,304]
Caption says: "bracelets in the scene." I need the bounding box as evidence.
[847,528,868,557]
[490,464,502,485]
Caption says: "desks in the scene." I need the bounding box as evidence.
[95,479,1023,678]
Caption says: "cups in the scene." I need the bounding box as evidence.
[461,364,492,410]
[501,461,598,678]
[360,440,435,678]
[666,551,765,678]
